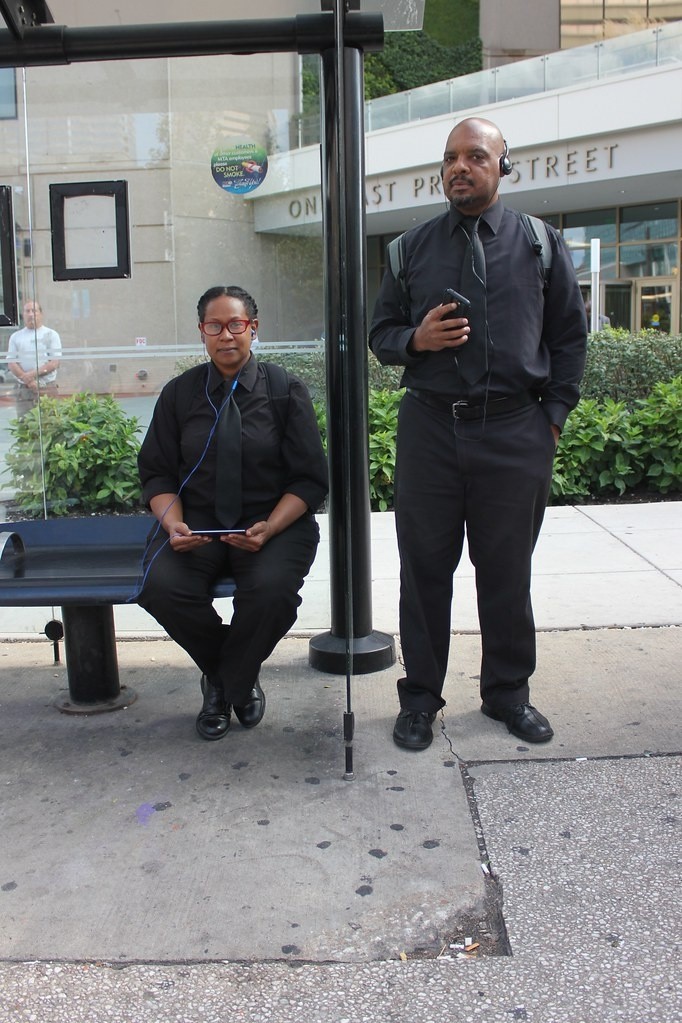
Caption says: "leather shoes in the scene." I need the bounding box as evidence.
[195,674,232,740]
[481,702,554,742]
[393,708,437,749]
[233,676,265,728]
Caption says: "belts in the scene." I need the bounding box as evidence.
[406,388,535,421]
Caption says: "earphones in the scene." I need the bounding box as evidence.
[251,330,255,338]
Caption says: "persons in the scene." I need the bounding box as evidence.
[137,285,329,740]
[368,118,587,750]
[8,302,63,487]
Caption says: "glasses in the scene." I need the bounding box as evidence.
[200,319,251,336]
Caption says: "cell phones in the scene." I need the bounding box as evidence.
[189,530,246,542]
[440,289,470,351]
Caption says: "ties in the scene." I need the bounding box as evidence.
[214,381,242,529]
[458,216,488,386]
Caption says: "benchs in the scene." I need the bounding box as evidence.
[0,517,243,714]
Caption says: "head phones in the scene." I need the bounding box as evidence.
[440,139,513,180]
